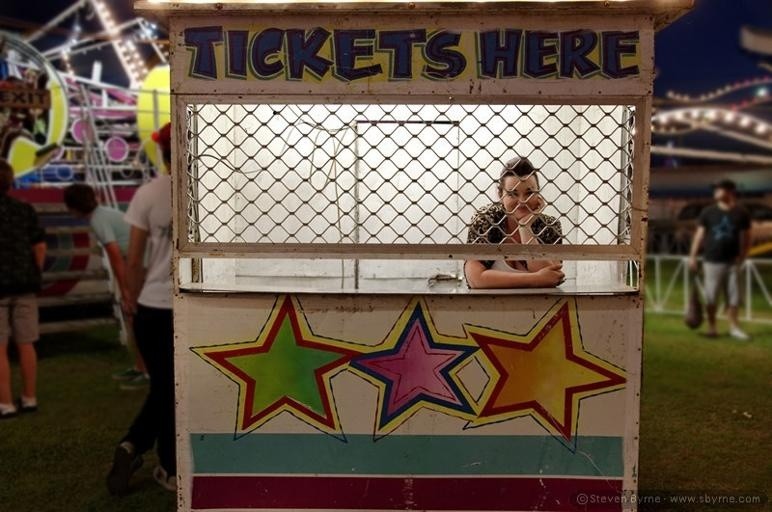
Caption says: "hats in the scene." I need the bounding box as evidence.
[150,118,172,146]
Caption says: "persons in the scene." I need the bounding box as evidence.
[688,184,751,343]
[108,122,195,495]
[0,166,46,419]
[61,182,150,390]
[666,203,701,248]
[463,158,568,288]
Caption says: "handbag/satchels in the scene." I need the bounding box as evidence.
[685,269,704,329]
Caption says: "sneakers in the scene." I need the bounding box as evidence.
[19,395,39,412]
[0,401,17,417]
[150,462,177,492]
[106,440,144,494]
[112,366,139,381]
[122,374,151,391]
